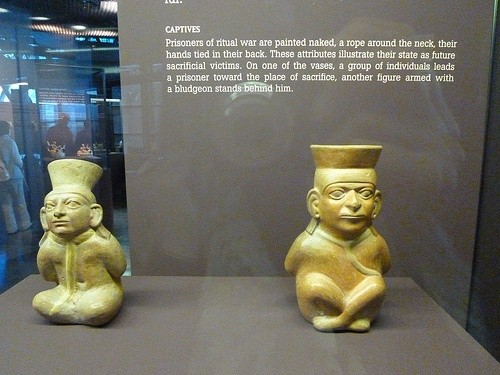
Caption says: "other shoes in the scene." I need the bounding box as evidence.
[9,228,20,235]
[23,222,33,232]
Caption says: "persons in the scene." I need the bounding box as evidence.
[283,146,393,333]
[43,111,75,157]
[95,112,108,149]
[32,159,127,326]
[0,120,33,234]
[75,117,93,155]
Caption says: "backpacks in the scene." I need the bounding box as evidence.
[0,160,10,182]
